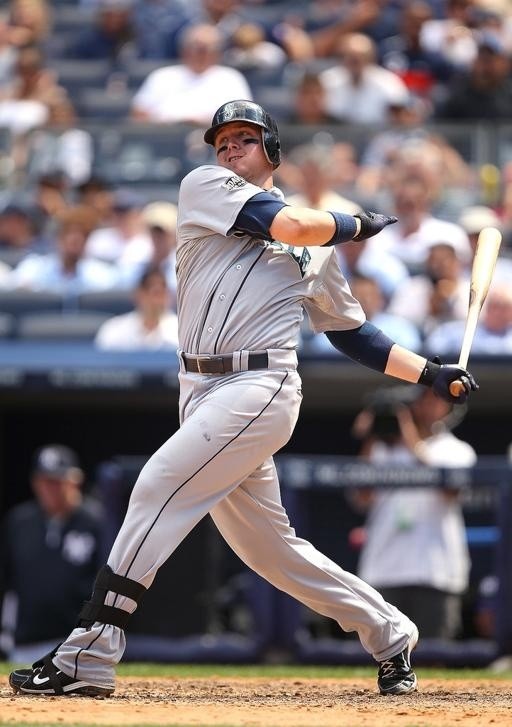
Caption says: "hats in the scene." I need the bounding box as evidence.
[137,200,179,234]
[31,445,81,479]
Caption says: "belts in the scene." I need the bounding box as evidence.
[181,349,269,375]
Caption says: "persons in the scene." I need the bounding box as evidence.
[0,0,512,675]
[9,101,478,698]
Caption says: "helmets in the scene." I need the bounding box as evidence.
[202,98,281,171]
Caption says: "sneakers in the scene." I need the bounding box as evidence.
[8,660,116,697]
[377,619,420,695]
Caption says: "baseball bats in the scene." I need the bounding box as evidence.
[450,227,503,397]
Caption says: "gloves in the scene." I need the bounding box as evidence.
[353,210,398,243]
[432,364,480,405]
